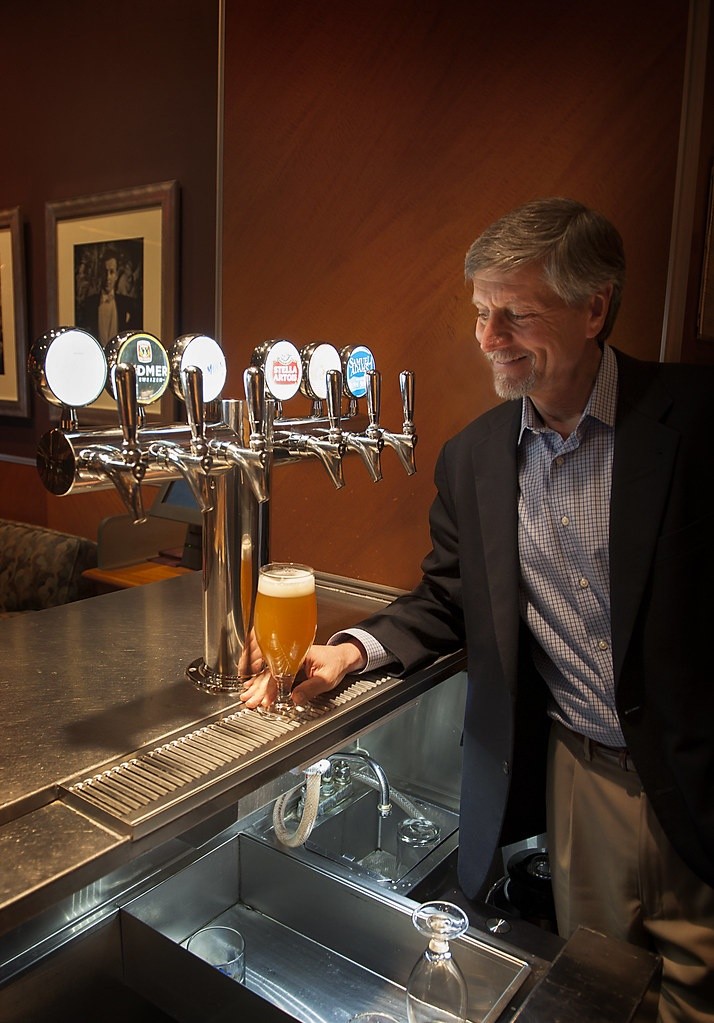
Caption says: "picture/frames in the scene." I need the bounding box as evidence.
[0,177,180,431]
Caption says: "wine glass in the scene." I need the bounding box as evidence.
[406,901,468,1023]
[253,563,317,719]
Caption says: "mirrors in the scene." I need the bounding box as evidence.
[0,0,226,466]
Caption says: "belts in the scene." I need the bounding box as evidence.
[553,725,636,772]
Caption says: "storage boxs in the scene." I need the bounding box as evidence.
[117,832,528,1023]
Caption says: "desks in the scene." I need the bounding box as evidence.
[80,544,211,596]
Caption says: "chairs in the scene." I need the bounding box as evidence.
[0,517,98,616]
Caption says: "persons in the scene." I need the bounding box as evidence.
[77,249,140,350]
[239,197,714,1023]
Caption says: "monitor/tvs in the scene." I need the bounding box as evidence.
[148,479,204,571]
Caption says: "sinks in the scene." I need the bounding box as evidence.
[126,830,533,1022]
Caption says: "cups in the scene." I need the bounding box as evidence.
[347,1013,400,1023]
[398,818,441,879]
[187,926,246,986]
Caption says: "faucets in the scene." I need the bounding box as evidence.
[307,370,345,491]
[327,753,391,822]
[229,365,271,504]
[348,370,385,484]
[102,361,148,526]
[167,367,214,514]
[304,781,459,884]
[386,369,417,476]
